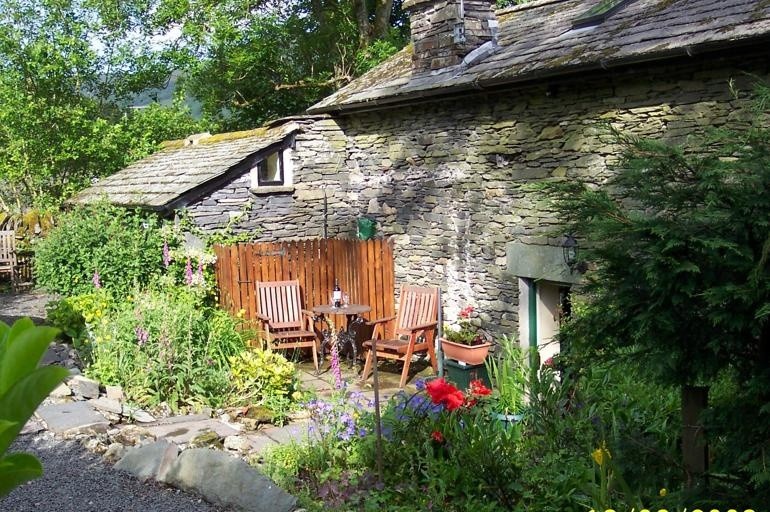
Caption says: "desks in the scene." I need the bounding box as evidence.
[310,302,373,376]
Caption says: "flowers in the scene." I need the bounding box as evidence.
[444,303,489,346]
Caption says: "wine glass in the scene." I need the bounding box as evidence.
[327,289,350,310]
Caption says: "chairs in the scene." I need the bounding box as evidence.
[253,277,321,369]
[361,284,443,391]
[0,229,20,294]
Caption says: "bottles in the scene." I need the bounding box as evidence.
[333,278,341,308]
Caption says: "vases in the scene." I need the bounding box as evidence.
[438,336,493,367]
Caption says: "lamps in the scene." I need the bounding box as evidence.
[561,231,589,275]
